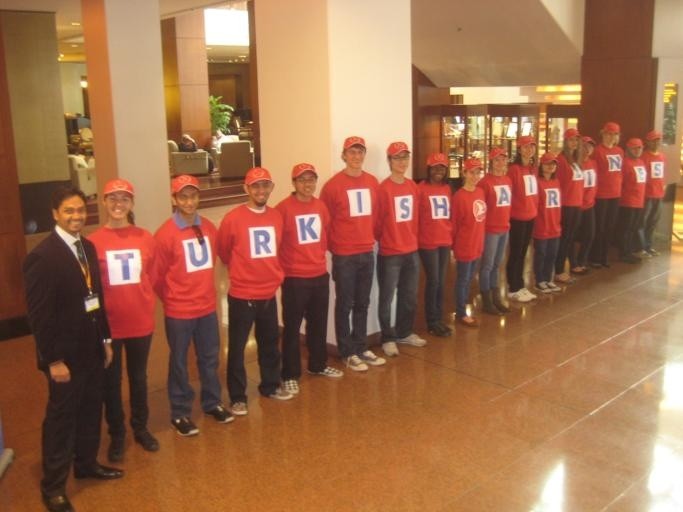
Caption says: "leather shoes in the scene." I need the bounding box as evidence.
[47,495,72,512]
[74,465,123,480]
[455,314,481,327]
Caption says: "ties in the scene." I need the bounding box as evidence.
[73,239,91,288]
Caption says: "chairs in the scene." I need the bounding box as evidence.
[64,114,254,203]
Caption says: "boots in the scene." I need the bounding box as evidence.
[481,290,504,316]
[489,286,512,313]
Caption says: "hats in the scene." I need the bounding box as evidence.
[172,175,199,193]
[463,159,483,172]
[291,163,316,178]
[426,152,448,167]
[103,179,134,197]
[488,123,663,164]
[244,167,272,186]
[387,141,410,156]
[344,136,365,150]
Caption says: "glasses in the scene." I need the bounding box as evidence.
[391,155,409,161]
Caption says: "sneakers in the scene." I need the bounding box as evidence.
[342,321,450,372]
[170,416,199,436]
[268,387,293,401]
[108,436,122,461]
[506,247,660,302]
[231,402,248,415]
[205,405,235,424]
[135,432,158,452]
[284,380,298,395]
[307,366,343,378]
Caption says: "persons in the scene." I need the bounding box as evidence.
[86,178,160,465]
[178,133,214,172]
[24,184,123,512]
[151,174,237,437]
[452,158,488,327]
[319,135,387,372]
[376,142,427,359]
[506,121,668,303]
[219,166,296,416]
[473,147,513,316]
[272,161,344,395]
[417,152,455,337]
